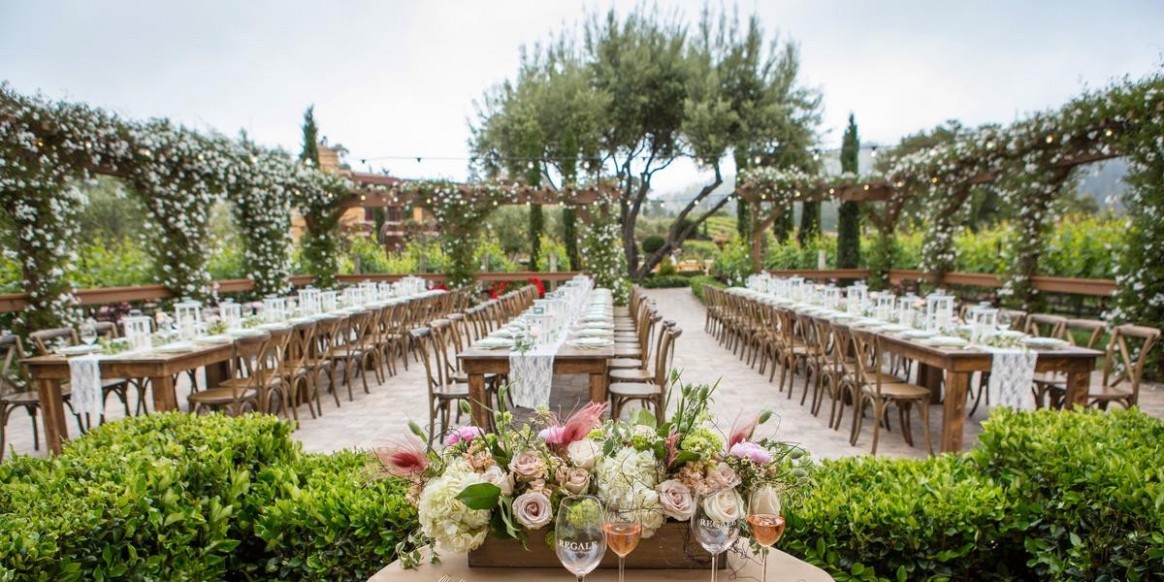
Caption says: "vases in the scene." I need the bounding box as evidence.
[468,521,727,569]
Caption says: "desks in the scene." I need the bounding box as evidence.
[363,539,836,582]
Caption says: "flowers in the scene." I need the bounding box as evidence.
[354,365,813,571]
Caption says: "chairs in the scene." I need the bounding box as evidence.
[0,272,1161,455]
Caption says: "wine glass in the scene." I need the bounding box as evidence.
[551,491,609,581]
[689,484,742,582]
[605,486,643,582]
[748,480,785,582]
[79,322,99,357]
